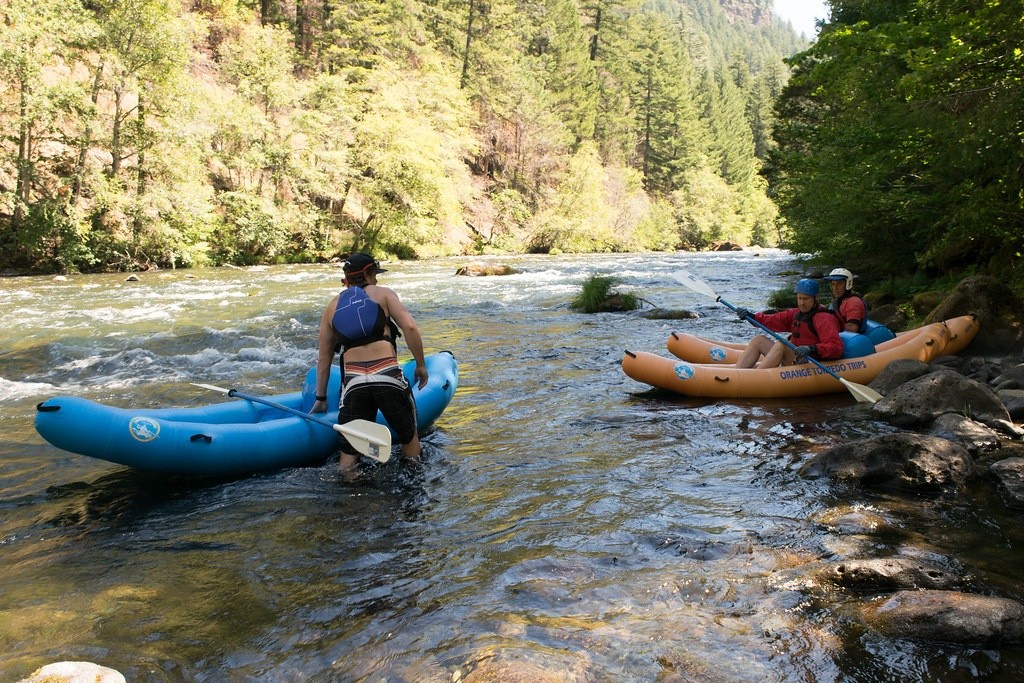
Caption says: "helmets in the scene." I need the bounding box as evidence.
[822,268,853,290]
[794,278,820,298]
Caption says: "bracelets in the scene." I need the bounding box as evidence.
[315,394,327,401]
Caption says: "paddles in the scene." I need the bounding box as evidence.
[187,380,393,467]
[671,267,885,403]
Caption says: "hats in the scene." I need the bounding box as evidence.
[343,252,388,276]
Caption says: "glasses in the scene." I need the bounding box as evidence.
[347,259,380,276]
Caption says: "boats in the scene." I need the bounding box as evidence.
[621,315,952,397]
[34,348,460,478]
[667,313,982,364]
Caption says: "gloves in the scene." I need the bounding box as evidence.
[794,345,816,359]
[737,307,755,320]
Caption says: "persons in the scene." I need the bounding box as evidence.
[733,278,844,369]
[822,268,868,334]
[307,253,430,483]
